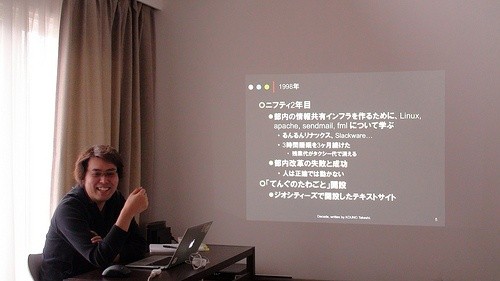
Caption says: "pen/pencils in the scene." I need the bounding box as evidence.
[163,244,178,249]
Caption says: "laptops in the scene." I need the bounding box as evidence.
[124,221,213,268]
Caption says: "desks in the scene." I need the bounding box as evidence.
[62,244,255,281]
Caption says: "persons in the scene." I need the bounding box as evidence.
[39,145,150,281]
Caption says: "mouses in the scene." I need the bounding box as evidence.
[101,265,131,278]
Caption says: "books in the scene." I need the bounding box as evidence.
[150,242,209,254]
